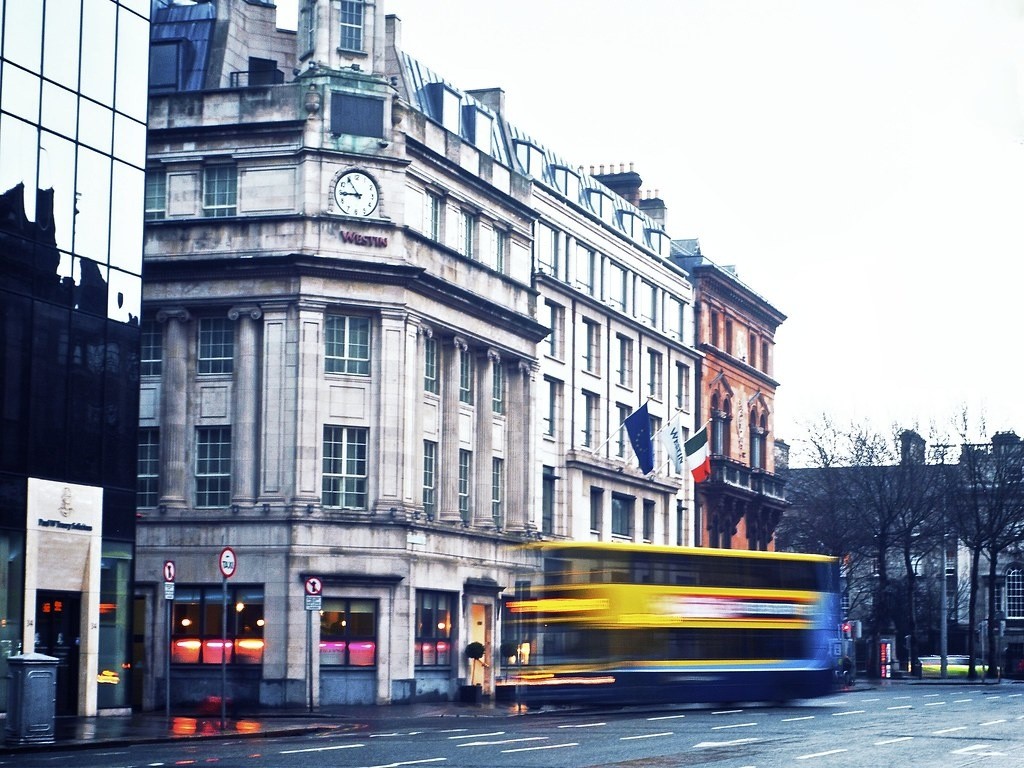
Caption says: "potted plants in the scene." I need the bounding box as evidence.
[461,642,486,704]
[495,642,520,703]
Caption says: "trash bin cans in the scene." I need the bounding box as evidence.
[3,653,62,751]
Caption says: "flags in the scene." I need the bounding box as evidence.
[683,425,711,483]
[660,414,686,474]
[624,402,654,476]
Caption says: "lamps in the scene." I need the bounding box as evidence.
[308,505,314,513]
[160,505,166,513]
[527,529,533,537]
[263,504,269,512]
[391,508,397,518]
[428,512,434,521]
[414,510,421,520]
[497,524,503,532]
[231,505,238,512]
[538,532,543,538]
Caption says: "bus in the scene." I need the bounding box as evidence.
[499,539,839,714]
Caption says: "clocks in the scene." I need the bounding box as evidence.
[335,169,379,217]
